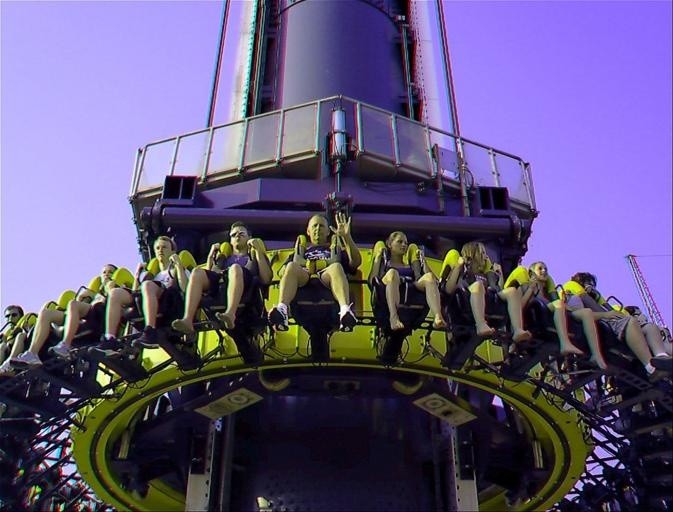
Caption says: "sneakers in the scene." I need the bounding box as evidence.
[130,325,160,349]
[9,350,44,371]
[0,363,15,378]
[86,333,121,361]
[267,303,290,331]
[46,340,71,363]
[645,353,673,385]
[338,305,357,333]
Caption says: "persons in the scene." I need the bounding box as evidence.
[444,241,532,344]
[171,221,271,332]
[1,305,38,374]
[368,231,447,330]
[557,424,672,511]
[268,211,362,332]
[8,264,118,368]
[516,261,673,435]
[15,447,116,511]
[87,236,191,359]
